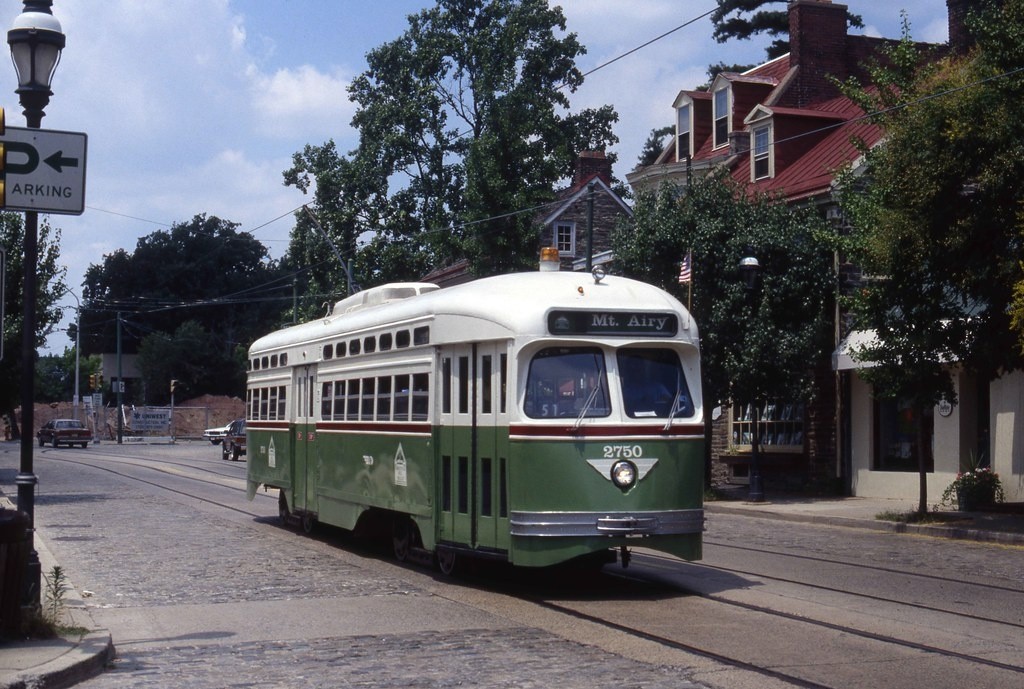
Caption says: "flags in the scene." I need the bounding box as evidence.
[678,253,691,282]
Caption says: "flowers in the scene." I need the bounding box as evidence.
[932,448,1006,513]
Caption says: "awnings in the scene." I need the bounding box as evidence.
[830,316,987,370]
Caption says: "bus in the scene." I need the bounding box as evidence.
[246,248,708,583]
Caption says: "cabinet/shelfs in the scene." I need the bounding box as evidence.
[730,396,805,450]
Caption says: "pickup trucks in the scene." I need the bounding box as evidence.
[222,419,246,460]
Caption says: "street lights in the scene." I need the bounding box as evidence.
[738,257,765,501]
[5,1,67,636]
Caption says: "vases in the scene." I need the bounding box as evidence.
[956,487,995,514]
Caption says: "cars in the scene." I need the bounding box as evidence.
[204,420,236,446]
[36,419,91,448]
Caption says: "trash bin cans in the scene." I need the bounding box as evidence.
[0,508,34,636]
